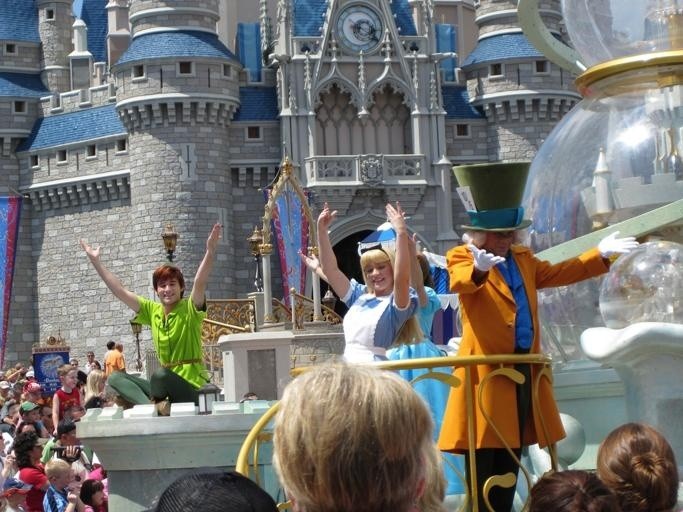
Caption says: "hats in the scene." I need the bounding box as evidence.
[0,381,11,390]
[57,416,79,436]
[19,400,41,416]
[448,160,534,231]
[25,371,34,377]
[4,478,34,497]
[14,431,49,451]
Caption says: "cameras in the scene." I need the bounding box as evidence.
[49,447,82,461]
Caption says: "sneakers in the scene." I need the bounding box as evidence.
[156,401,170,417]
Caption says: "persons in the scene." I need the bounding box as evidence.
[436,231,638,512]
[314,200,411,380]
[297,232,471,495]
[79,221,224,418]
[1,336,128,512]
[271,362,447,512]
[141,470,279,512]
[528,469,619,510]
[596,422,679,512]
[243,392,258,401]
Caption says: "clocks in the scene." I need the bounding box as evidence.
[333,0,387,55]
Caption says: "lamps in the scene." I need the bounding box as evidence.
[196,371,222,415]
[161,224,180,261]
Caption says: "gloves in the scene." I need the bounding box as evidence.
[468,244,506,273]
[598,230,639,260]
